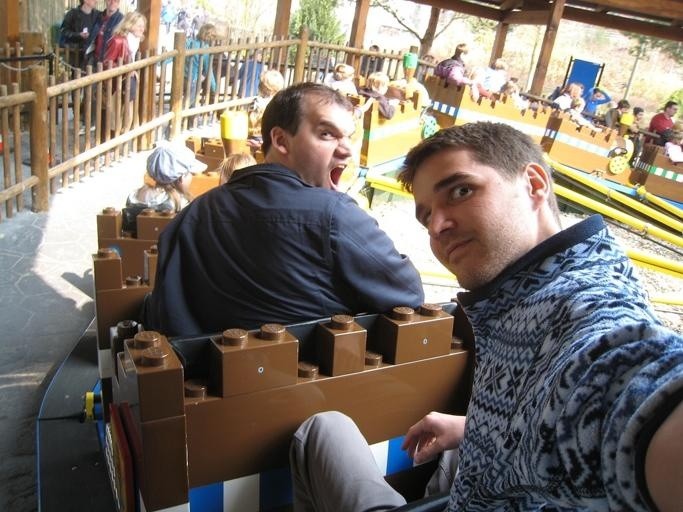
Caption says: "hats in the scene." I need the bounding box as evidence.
[147,144,206,185]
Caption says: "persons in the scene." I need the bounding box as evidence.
[218,43,407,175]
[218,150,258,183]
[288,118,683,512]
[434,43,683,150]
[59,0,269,140]
[129,140,209,206]
[133,81,425,374]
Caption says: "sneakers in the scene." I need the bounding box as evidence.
[79,126,96,136]
